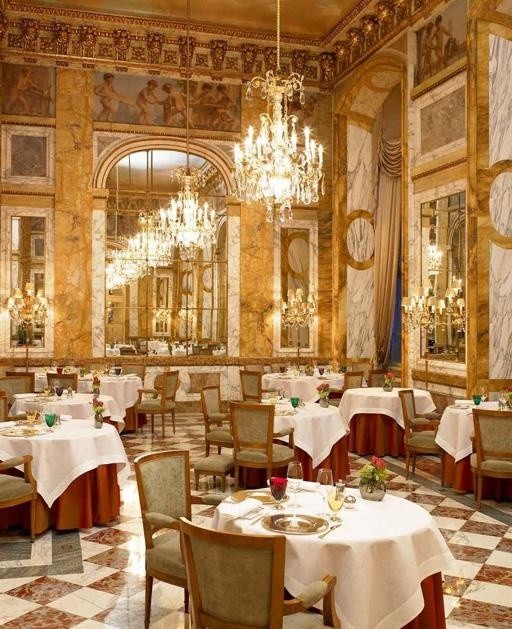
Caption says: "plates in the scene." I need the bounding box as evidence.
[262,514,329,535]
[230,489,289,504]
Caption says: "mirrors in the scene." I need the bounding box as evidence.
[10,216,48,349]
[418,190,471,365]
[103,149,226,356]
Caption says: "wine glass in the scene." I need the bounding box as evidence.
[43,383,76,401]
[267,476,289,511]
[24,407,56,435]
[269,394,299,415]
[472,384,489,410]
[287,461,306,509]
[316,467,333,517]
[325,488,346,523]
[287,364,334,380]
[56,364,87,378]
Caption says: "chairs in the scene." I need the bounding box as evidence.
[119,347,135,356]
[192,343,220,356]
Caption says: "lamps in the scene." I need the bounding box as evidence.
[437,286,466,332]
[401,293,444,334]
[229,1,328,226]
[149,153,222,262]
[1,290,48,331]
